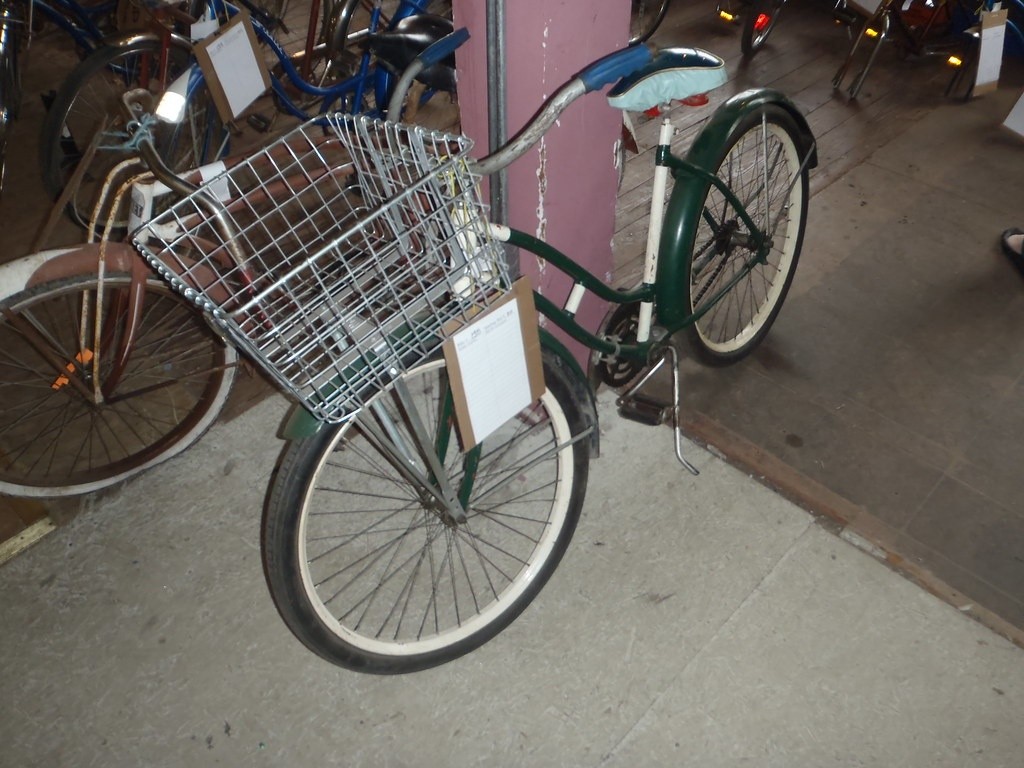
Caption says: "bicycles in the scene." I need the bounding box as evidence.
[130,27,818,675]
[0,0,458,498]
[629,0,1024,102]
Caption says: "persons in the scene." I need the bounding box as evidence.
[1002,228,1024,273]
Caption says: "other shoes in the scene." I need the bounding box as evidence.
[1001,227,1024,268]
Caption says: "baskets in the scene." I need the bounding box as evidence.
[128,109,514,425]
